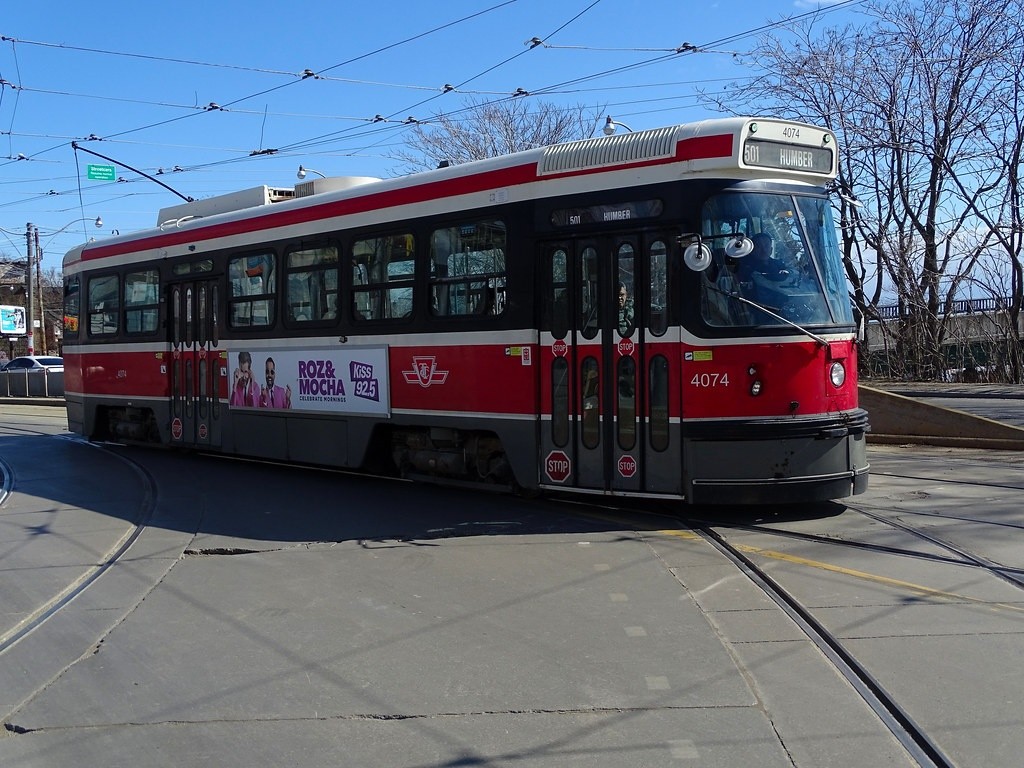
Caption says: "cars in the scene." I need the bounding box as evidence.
[0,354,65,373]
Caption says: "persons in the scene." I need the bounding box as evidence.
[230,352,260,407]
[474,286,495,315]
[619,282,662,335]
[734,233,800,324]
[259,357,292,409]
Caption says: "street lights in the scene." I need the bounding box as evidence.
[0,217,104,356]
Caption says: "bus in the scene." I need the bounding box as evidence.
[55,113,874,512]
